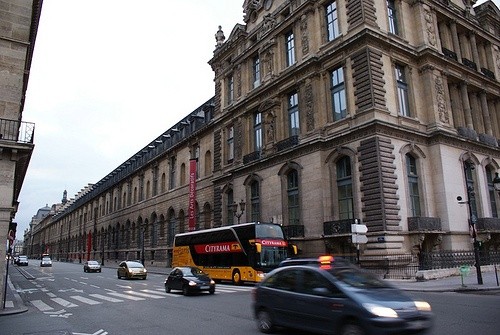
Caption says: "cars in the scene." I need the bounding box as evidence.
[17,257,28,266]
[116,260,147,280]
[13,256,28,264]
[40,258,52,267]
[164,266,216,297]
[83,261,102,273]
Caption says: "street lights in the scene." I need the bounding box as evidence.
[456,184,484,285]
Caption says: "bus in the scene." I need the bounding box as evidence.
[172,221,298,286]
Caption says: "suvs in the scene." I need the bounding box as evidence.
[251,256,434,335]
